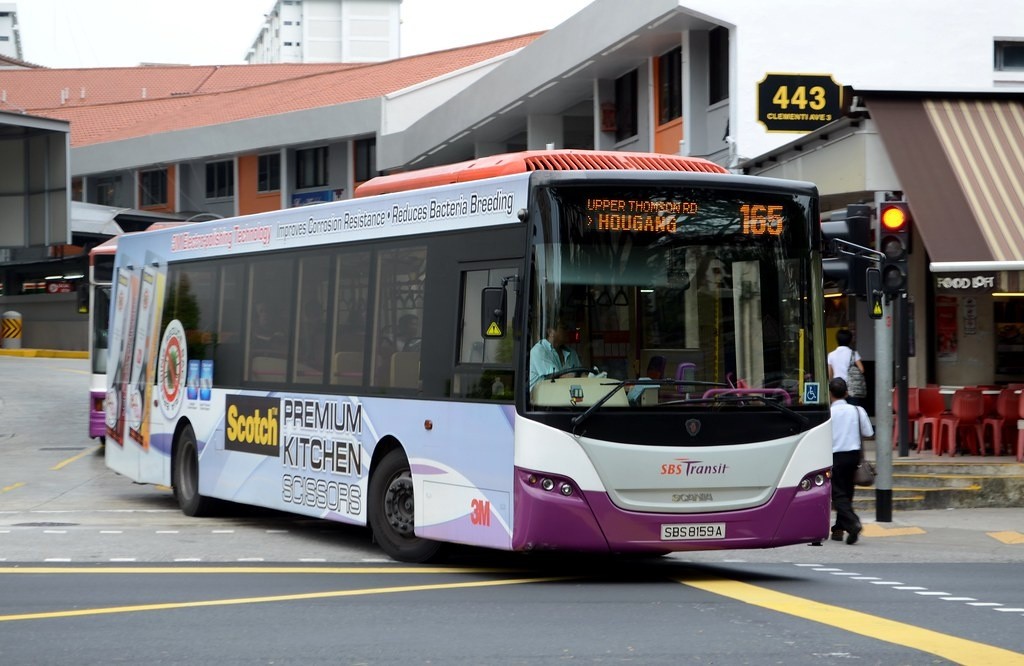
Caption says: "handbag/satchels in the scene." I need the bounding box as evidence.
[847,350,866,397]
[856,463,876,486]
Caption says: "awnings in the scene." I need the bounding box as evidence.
[865,94,1024,296]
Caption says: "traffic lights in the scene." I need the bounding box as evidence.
[877,202,914,301]
[820,204,870,295]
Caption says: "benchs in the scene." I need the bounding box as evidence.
[252,356,322,383]
[639,349,705,390]
[390,352,420,389]
[334,352,382,385]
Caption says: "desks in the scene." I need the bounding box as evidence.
[890,386,1021,394]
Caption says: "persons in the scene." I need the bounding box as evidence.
[530,310,587,395]
[381,314,421,362]
[827,328,864,406]
[300,299,325,368]
[829,377,874,545]
[252,309,284,344]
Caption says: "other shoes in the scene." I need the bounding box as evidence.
[832,531,844,542]
[847,522,861,544]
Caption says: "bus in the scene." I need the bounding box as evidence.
[89,145,886,561]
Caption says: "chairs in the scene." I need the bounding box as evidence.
[893,383,1024,462]
